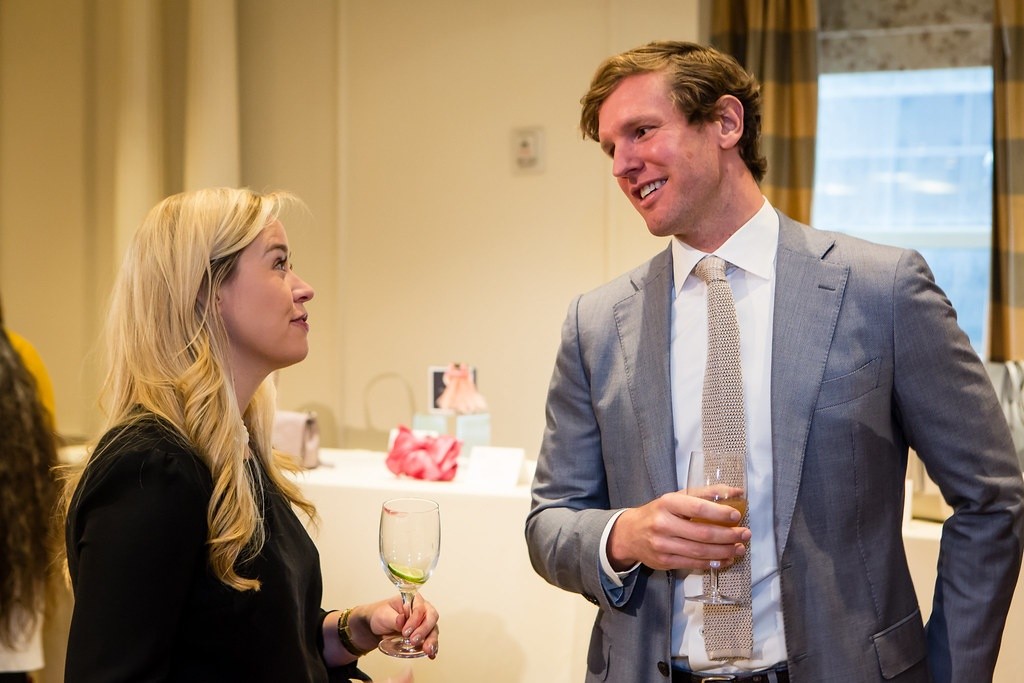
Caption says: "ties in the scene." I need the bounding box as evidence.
[691,256,755,661]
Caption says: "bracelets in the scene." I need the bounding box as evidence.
[337,607,368,656]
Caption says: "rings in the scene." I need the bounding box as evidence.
[710,560,720,569]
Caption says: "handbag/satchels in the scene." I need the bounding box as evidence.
[271,408,320,469]
[385,425,461,481]
[913,359,1024,523]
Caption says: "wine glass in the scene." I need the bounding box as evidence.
[682,451,751,606]
[378,499,440,658]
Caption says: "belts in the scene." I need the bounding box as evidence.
[671,664,790,683]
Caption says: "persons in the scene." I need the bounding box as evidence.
[0,295,69,683]
[63,191,439,683]
[525,41,1024,683]
